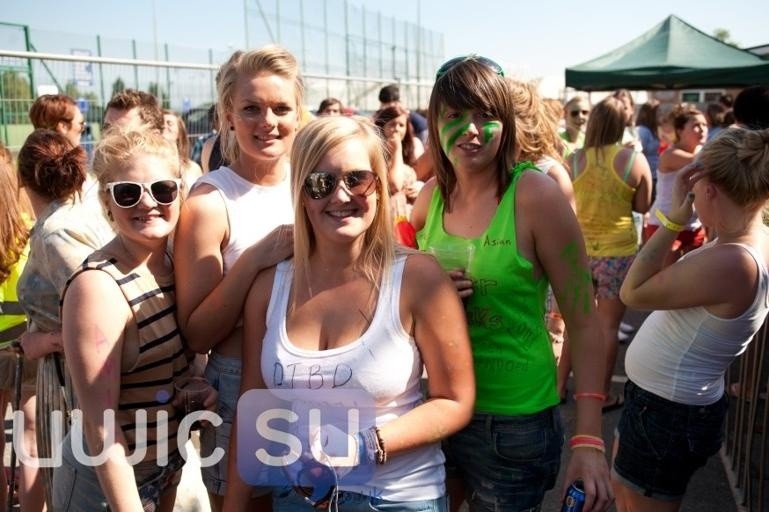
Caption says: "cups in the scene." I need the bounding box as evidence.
[174,375,213,430]
[430,242,475,280]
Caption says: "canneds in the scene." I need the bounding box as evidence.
[560,479,586,512]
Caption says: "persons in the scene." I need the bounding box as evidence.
[0,47,769,512]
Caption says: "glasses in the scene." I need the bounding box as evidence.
[268,456,337,504]
[105,178,181,210]
[304,170,379,200]
[435,56,504,79]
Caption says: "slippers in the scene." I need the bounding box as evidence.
[602,394,624,415]
